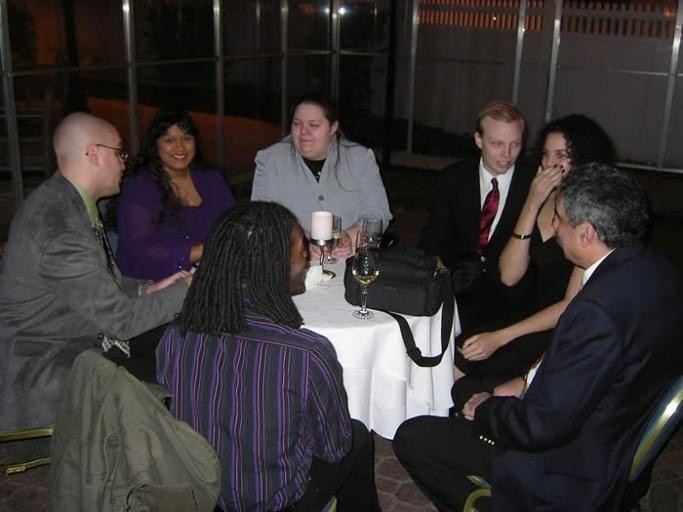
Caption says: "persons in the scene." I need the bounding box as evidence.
[153,201,386,511]
[418,101,535,323]
[110,110,237,277]
[248,96,393,260]
[0,109,196,435]
[451,113,619,385]
[391,162,678,511]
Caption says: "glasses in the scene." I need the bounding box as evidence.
[85,144,128,163]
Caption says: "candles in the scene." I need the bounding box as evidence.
[310,211,333,241]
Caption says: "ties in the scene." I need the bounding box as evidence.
[93,213,130,359]
[476,177,499,257]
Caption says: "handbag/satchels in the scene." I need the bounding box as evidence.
[344,244,450,318]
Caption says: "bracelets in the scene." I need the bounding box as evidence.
[513,227,535,239]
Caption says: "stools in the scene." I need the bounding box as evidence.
[0,428,55,478]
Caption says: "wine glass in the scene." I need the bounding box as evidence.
[323,218,341,265]
[351,250,380,320]
[361,216,382,234]
[354,230,377,252]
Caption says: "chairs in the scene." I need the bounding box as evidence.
[461,378,683,512]
[69,350,344,511]
